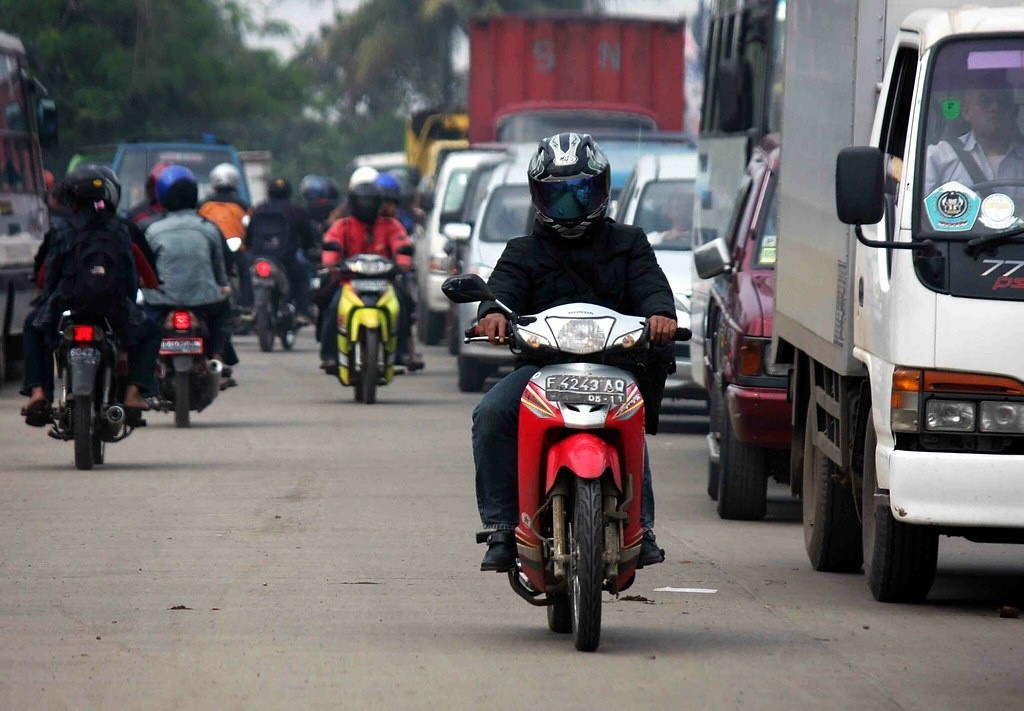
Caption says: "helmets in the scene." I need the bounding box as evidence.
[155,166,199,208]
[209,163,241,191]
[302,166,415,222]
[528,133,611,238]
[268,177,291,197]
[60,163,122,212]
[145,162,172,199]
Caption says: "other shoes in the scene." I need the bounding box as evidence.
[320,360,337,369]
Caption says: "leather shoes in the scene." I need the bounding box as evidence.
[638,531,663,566]
[480,530,516,570]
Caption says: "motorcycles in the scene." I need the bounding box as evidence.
[138,311,237,430]
[22,299,147,472]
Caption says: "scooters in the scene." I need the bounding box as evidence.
[241,214,310,352]
[315,241,419,407]
[440,273,696,655]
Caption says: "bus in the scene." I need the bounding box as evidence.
[687,0,782,393]
[0,29,60,386]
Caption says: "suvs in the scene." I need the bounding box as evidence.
[692,130,796,522]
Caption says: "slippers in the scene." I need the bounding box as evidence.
[20,401,44,416]
[220,377,236,389]
[124,401,151,412]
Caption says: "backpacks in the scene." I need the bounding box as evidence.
[60,215,125,316]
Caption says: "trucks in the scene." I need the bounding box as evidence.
[463,15,694,145]
[770,0,1023,603]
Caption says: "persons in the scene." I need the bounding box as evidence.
[480,133,678,572]
[78,162,435,390]
[21,165,158,415]
[920,76,1024,239]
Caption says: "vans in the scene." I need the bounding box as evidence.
[70,133,271,240]
[353,103,684,391]
[605,151,702,424]
